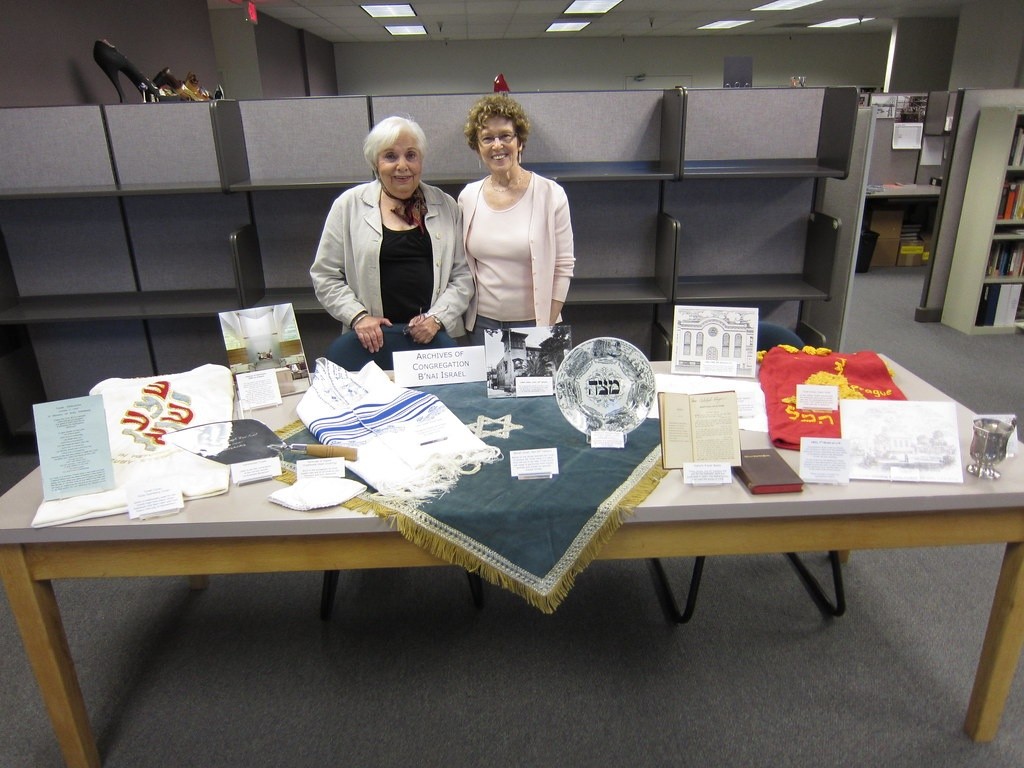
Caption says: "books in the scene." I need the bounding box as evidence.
[657,390,742,470]
[985,243,1024,278]
[1008,229,1024,234]
[1009,126,1024,166]
[734,448,804,495]
[975,283,1022,326]
[901,219,922,241]
[998,179,1024,219]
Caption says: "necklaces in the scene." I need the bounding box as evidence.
[491,167,522,192]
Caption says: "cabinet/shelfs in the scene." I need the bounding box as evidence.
[941,104,1024,336]
[0,86,877,457]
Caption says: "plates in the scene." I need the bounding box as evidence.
[556,337,655,435]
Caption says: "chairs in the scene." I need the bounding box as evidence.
[654,321,848,624]
[315,324,485,620]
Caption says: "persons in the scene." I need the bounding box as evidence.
[310,116,475,353]
[457,95,574,346]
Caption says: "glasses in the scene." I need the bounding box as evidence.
[477,132,518,148]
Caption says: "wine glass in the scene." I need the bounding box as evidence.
[967,418,1014,480]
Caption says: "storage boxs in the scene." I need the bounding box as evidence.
[897,240,924,267]
[868,239,900,266]
[870,210,905,238]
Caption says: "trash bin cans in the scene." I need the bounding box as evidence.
[855,229,880,273]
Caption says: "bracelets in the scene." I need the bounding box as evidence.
[352,312,369,329]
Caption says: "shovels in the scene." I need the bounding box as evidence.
[161,420,358,464]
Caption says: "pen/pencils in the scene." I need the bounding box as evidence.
[420,435,447,445]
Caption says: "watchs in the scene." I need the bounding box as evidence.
[431,316,442,328]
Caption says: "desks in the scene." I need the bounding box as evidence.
[0,351,1024,766]
[865,185,941,201]
